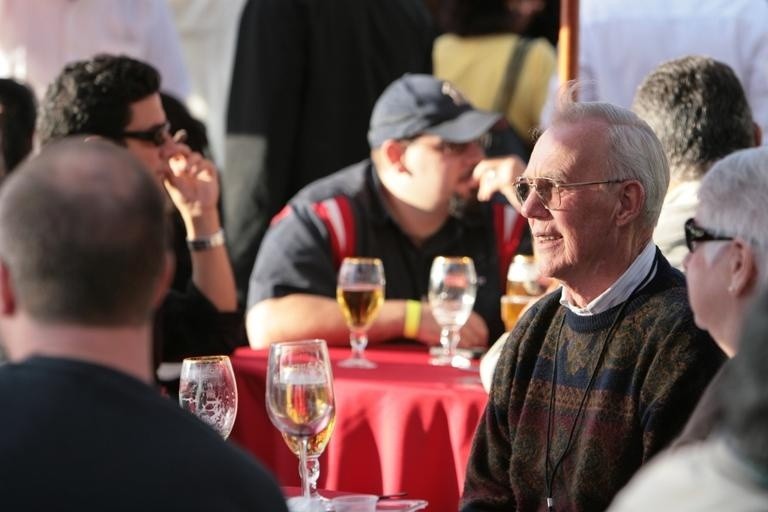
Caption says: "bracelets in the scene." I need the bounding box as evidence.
[400,296,425,343]
[184,230,228,253]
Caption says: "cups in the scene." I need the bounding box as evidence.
[179,353,239,444]
[333,493,379,512]
[500,255,546,338]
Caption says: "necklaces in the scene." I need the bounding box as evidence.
[544,245,660,512]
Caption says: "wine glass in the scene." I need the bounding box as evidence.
[335,255,386,369]
[263,337,336,512]
[427,256,476,366]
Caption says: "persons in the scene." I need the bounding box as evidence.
[29,48,251,364]
[605,141,768,512]
[1,76,41,322]
[240,69,529,363]
[0,133,292,512]
[428,1,558,147]
[628,53,761,279]
[459,97,724,512]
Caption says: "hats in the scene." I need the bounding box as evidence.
[368,74,505,145]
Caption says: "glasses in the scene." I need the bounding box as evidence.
[684,218,759,253]
[511,177,630,211]
[121,121,171,147]
